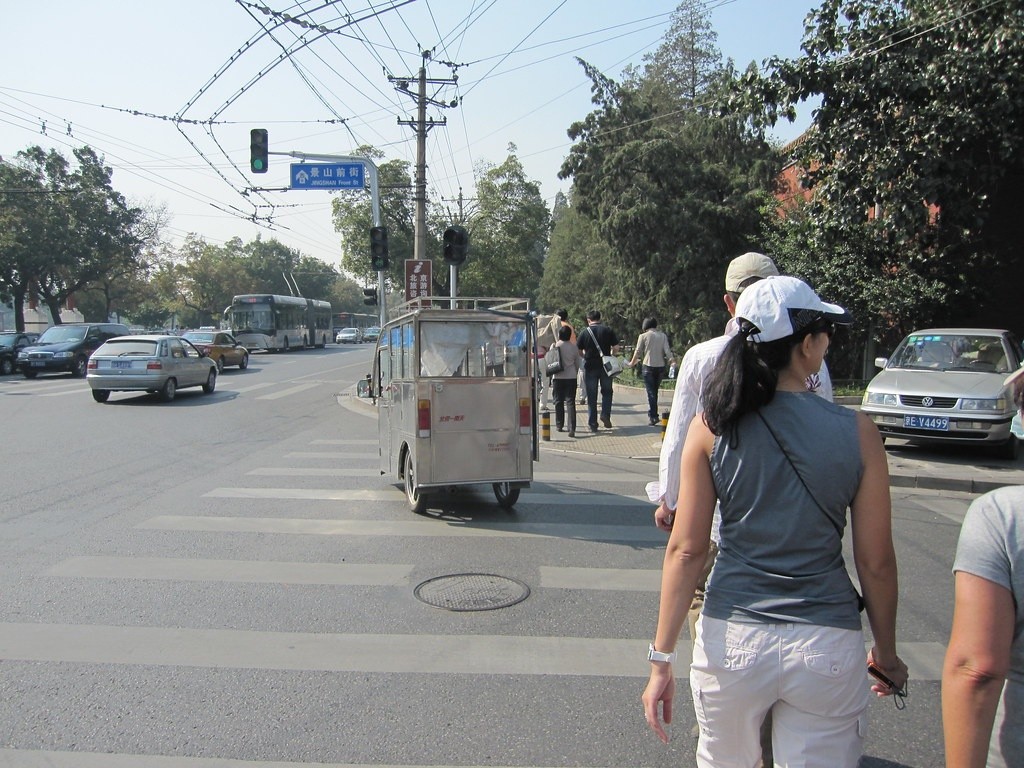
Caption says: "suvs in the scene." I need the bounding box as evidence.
[14,322,132,380]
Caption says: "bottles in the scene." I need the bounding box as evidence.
[668,366,675,378]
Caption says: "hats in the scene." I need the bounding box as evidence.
[725,252,780,293]
[734,276,844,343]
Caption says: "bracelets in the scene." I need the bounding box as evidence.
[871,646,899,671]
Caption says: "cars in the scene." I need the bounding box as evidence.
[362,328,382,342]
[86,333,220,403]
[175,325,250,374]
[859,326,1024,461]
[335,327,363,345]
[0,330,42,376]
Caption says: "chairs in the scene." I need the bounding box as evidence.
[921,341,956,364]
[976,350,1004,367]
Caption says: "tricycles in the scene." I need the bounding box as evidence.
[355,294,539,515]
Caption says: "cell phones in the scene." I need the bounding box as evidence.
[867,662,894,690]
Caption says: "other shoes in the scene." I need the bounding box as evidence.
[556,427,561,431]
[580,400,586,404]
[591,427,597,433]
[600,415,612,428]
[649,418,659,425]
[569,428,574,438]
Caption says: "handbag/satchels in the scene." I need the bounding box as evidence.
[544,344,564,377]
[603,355,623,378]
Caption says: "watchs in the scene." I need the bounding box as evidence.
[647,643,677,662]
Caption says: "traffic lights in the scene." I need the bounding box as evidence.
[443,225,469,265]
[364,288,377,305]
[369,227,389,271]
[250,128,269,173]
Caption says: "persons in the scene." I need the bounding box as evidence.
[628,316,676,426]
[646,252,833,762]
[640,274,909,768]
[941,366,1024,768]
[484,309,619,438]
[173,324,189,336]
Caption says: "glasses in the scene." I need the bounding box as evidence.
[787,319,838,344]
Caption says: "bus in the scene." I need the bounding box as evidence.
[222,269,333,355]
[332,311,380,344]
